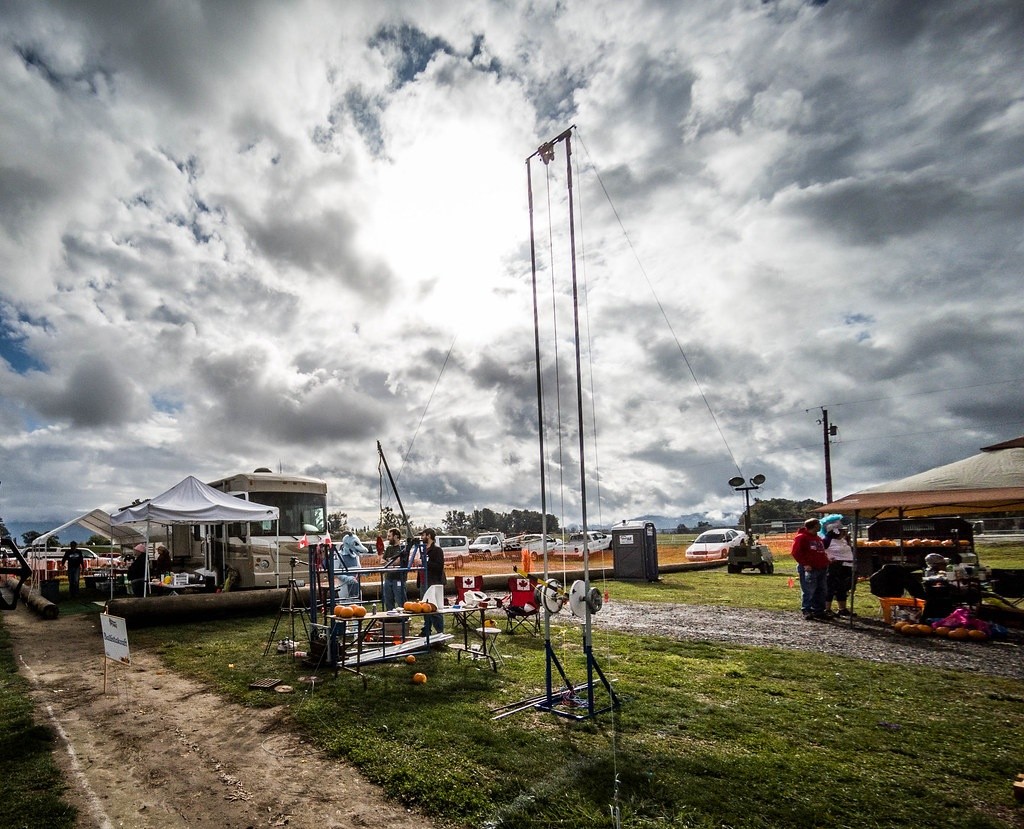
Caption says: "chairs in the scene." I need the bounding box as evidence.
[494,575,541,639]
[452,574,492,632]
[983,566,1024,617]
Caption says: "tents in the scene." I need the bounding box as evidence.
[811,434,1024,627]
[109,476,280,598]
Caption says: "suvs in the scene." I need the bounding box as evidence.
[413,536,470,568]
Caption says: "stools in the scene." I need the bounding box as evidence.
[476,626,507,667]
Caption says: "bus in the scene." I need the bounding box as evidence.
[167,468,332,589]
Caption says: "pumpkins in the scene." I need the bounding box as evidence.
[857,539,897,547]
[895,622,934,634]
[334,604,366,618]
[903,538,970,546]
[404,598,432,613]
[936,627,987,638]
[413,673,427,682]
[405,655,415,662]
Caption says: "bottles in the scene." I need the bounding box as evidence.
[292,651,307,657]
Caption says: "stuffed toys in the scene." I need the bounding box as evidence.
[333,533,369,632]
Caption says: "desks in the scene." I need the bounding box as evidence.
[322,602,500,674]
[147,581,207,595]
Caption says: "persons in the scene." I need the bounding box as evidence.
[380,529,409,612]
[412,528,447,637]
[127,544,173,598]
[60,541,85,603]
[791,514,857,621]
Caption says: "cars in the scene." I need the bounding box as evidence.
[467,531,563,561]
[329,539,405,571]
[685,529,746,561]
[0,545,123,570]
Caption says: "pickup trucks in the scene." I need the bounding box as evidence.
[551,531,613,557]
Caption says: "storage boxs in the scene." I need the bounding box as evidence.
[878,597,926,625]
[374,619,412,635]
